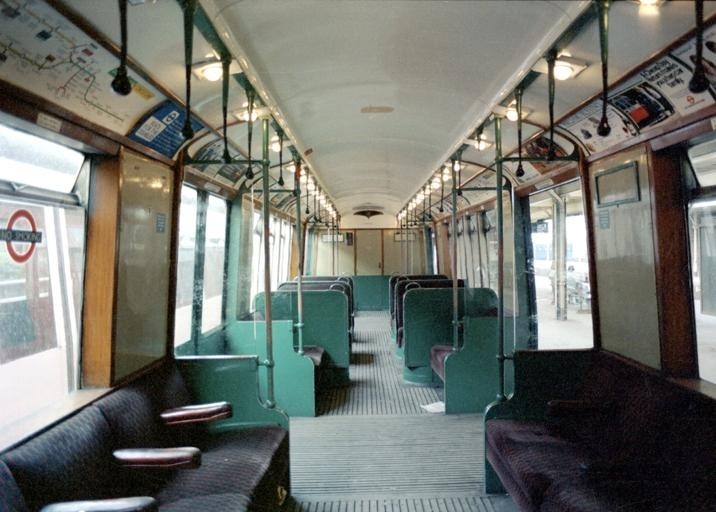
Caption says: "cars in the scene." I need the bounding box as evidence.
[548,237,591,312]
[530,233,577,276]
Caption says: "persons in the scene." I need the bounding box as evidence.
[565,265,576,302]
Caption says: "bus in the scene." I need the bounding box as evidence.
[0,128,289,449]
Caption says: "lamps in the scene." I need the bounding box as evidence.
[232,101,270,122]
[192,57,243,82]
[269,135,292,152]
[493,104,533,122]
[286,161,337,219]
[531,56,589,81]
[397,162,465,221]
[463,138,493,152]
